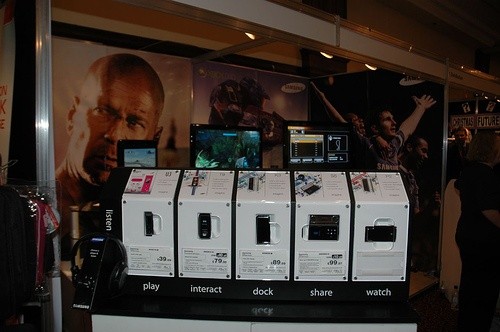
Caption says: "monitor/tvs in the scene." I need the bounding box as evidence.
[122,146,158,168]
[189,124,263,170]
[283,120,354,169]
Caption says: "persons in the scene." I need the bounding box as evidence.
[56,53,165,255]
[446,127,500,332]
[308,80,441,269]
